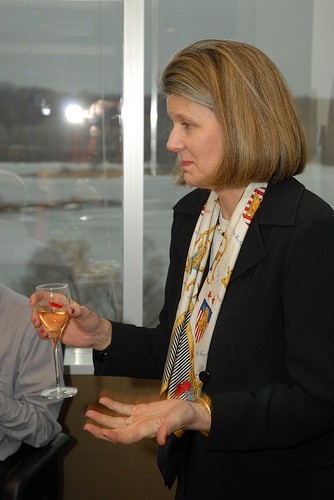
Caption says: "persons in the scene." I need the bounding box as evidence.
[28,40,334,499]
[0,284,64,500]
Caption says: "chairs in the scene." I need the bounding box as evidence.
[4,342,73,500]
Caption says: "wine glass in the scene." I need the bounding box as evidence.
[36,283,78,399]
